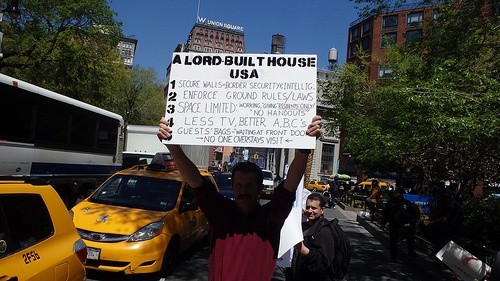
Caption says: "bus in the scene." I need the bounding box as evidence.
[0,74,130,184]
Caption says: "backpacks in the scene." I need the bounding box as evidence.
[307,218,354,281]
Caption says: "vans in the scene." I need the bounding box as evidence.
[261,169,277,197]
[349,181,389,195]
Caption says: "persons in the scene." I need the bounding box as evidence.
[381,189,419,264]
[366,180,381,215]
[329,176,338,210]
[157,116,322,281]
[349,183,359,195]
[323,190,331,208]
[342,182,351,194]
[294,192,334,281]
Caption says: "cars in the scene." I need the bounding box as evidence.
[215,172,237,203]
[0,180,89,281]
[69,151,221,281]
[304,179,327,192]
